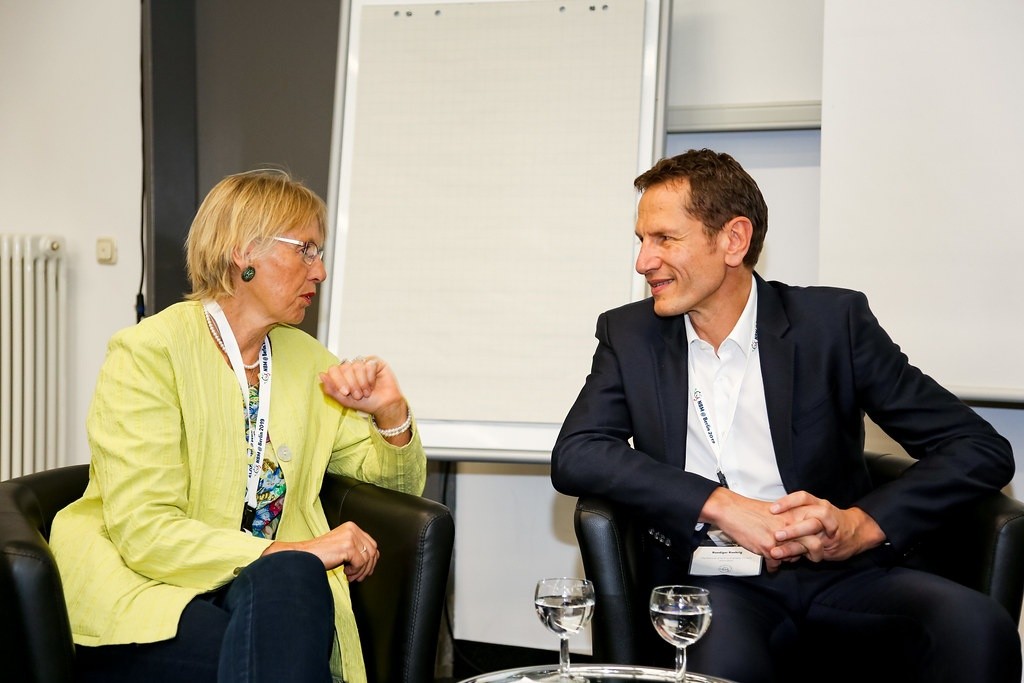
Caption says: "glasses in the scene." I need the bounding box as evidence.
[261,236,324,266]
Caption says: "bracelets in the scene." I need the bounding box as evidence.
[372,409,411,437]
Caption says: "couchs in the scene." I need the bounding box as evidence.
[0,464,456,683]
[574,451,1024,683]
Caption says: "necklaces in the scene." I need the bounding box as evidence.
[204,307,259,370]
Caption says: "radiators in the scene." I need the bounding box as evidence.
[0,235,69,480]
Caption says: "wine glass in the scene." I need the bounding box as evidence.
[649,585,712,683]
[534,578,596,683]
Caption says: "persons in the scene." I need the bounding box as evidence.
[549,150,1022,683]
[49,169,427,683]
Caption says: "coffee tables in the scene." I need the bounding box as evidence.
[460,663,734,683]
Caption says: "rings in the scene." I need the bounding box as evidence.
[359,546,368,553]
